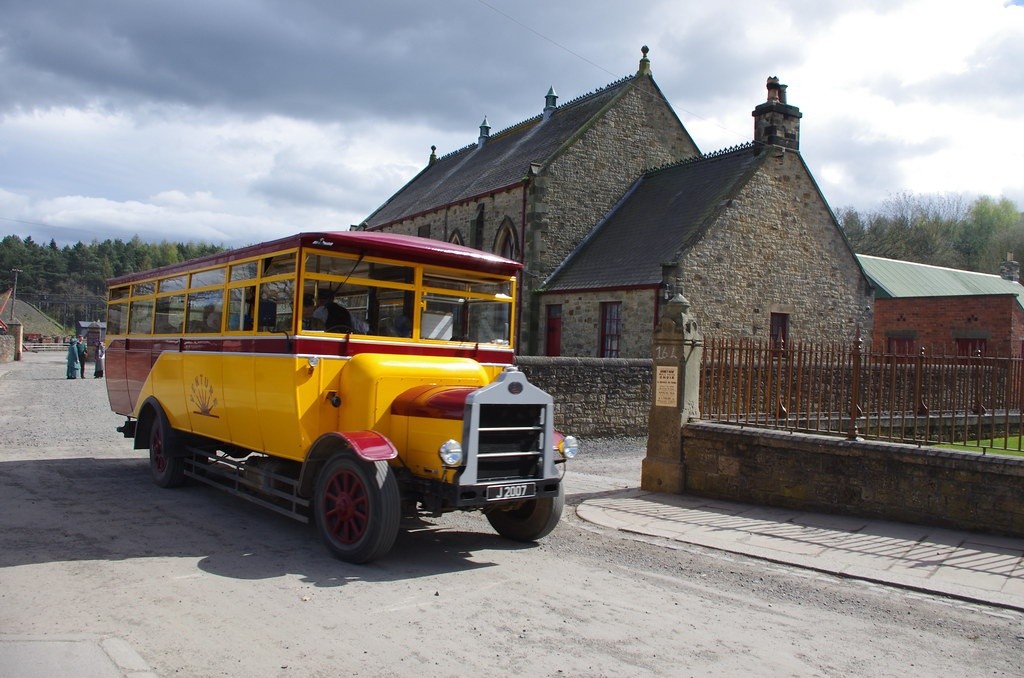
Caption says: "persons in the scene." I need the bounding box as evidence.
[244,288,277,326]
[65,338,81,379]
[93,340,104,378]
[313,288,369,334]
[76,334,88,379]
[277,293,326,332]
[203,303,215,322]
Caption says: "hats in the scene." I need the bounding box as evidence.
[70,338,77,343]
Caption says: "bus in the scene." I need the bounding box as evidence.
[105,231,579,563]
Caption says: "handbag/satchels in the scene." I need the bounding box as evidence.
[74,361,80,369]
[83,351,88,358]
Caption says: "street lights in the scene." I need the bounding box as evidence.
[11,268,23,322]
